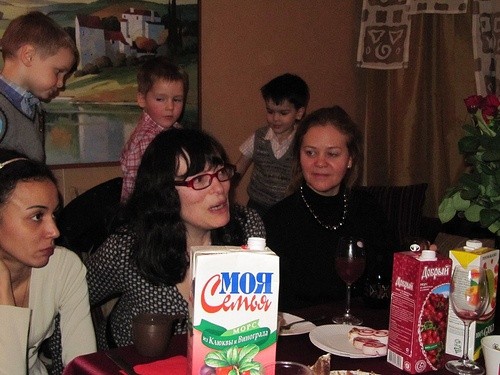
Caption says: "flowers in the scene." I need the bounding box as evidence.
[437,93,500,236]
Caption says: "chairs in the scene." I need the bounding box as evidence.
[55,177,145,259]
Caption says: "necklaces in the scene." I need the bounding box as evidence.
[299,185,349,231]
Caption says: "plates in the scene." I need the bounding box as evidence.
[309,324,388,358]
[278,312,316,336]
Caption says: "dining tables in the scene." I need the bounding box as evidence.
[62,304,500,375]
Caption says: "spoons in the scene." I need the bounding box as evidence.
[280,319,309,330]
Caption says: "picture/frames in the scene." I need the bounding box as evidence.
[0,0,203,170]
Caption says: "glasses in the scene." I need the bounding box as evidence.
[174,164,237,191]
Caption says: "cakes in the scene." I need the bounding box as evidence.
[309,352,331,375]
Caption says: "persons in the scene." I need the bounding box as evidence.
[0,149,98,375]
[0,10,79,164]
[122,60,188,205]
[262,104,389,325]
[231,73,311,219]
[52,127,266,375]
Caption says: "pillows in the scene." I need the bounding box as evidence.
[362,183,428,245]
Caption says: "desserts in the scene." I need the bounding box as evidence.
[348,328,388,356]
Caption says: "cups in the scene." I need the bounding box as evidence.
[132,314,174,357]
[259,360,314,375]
[480,335,500,375]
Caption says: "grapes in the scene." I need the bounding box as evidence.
[419,293,448,370]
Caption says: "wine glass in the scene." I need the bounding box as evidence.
[444,264,489,375]
[332,237,367,326]
